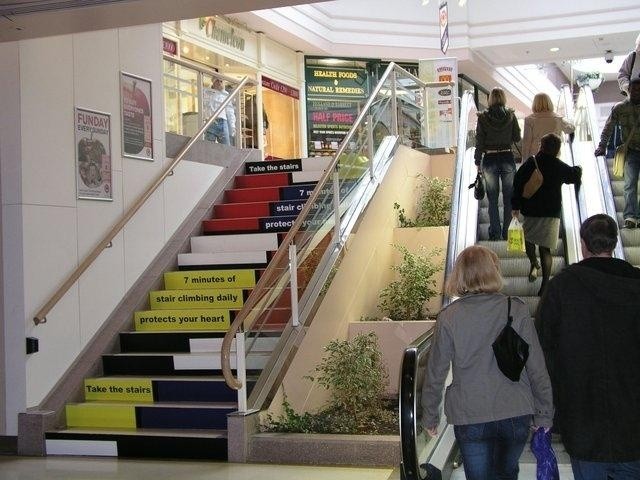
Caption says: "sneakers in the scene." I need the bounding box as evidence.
[625,217,637,229]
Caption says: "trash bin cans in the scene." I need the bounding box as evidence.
[183,111,199,137]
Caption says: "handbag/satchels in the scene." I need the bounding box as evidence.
[612,145,626,180]
[468,172,485,201]
[492,323,529,383]
[522,155,544,199]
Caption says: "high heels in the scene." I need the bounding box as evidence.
[538,284,547,297]
[529,260,541,283]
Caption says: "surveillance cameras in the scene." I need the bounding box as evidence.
[605,51,614,63]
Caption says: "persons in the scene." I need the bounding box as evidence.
[535,211,639,480]
[614,34,640,95]
[473,86,523,241]
[510,132,583,297]
[201,76,239,145]
[594,78,640,230]
[417,246,557,480]
[521,91,575,163]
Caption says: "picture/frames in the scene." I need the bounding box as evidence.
[73,70,154,202]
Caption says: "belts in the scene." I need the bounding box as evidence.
[485,149,514,154]
